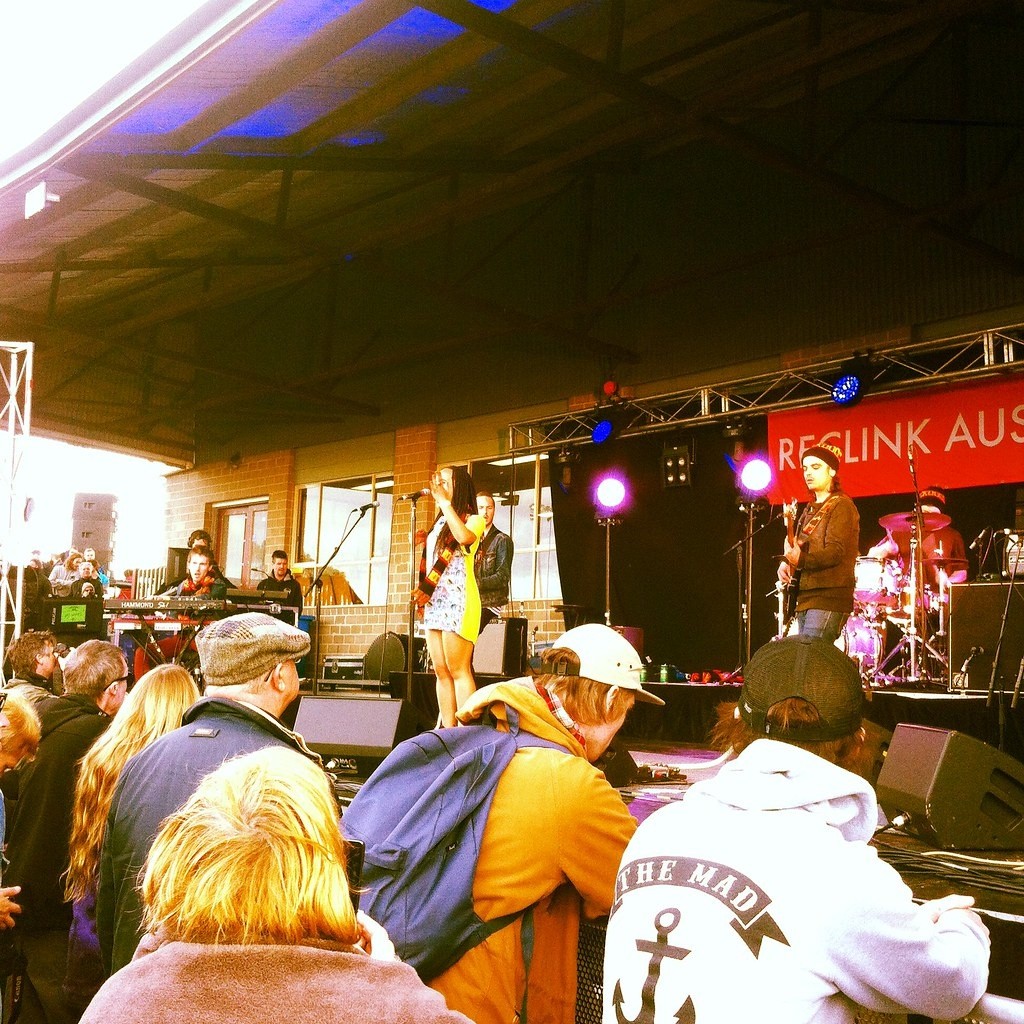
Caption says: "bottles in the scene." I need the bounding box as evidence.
[660,664,669,683]
[640,665,647,682]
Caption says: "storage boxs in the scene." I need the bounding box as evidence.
[472,616,528,676]
[323,654,370,691]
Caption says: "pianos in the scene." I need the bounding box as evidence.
[103,598,238,665]
[166,588,290,599]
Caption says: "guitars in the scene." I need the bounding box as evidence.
[781,499,802,626]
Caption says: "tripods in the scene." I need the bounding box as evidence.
[862,472,953,692]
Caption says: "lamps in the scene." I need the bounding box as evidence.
[661,435,696,490]
[832,352,875,407]
[24,179,60,219]
[591,418,613,443]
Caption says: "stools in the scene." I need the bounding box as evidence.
[182,650,201,686]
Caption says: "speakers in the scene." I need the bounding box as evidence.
[72,491,119,576]
[876,724,1024,854]
[471,618,529,677]
[948,581,1024,696]
[845,717,893,788]
[294,694,438,780]
[589,736,640,787]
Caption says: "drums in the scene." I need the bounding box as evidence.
[898,586,950,617]
[853,555,898,602]
[832,612,883,684]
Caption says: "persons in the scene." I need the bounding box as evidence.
[426,624,665,1024]
[601,634,991,1024]
[30,545,133,599]
[60,612,478,1024]
[0,629,129,1024]
[133,530,239,683]
[866,486,968,677]
[257,550,303,626]
[424,466,514,728]
[778,445,860,644]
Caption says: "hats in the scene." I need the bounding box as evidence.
[532,623,667,707]
[921,486,947,513]
[800,443,842,472]
[738,635,864,741]
[195,612,312,686]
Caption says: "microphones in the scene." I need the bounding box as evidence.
[908,451,914,474]
[400,489,431,500]
[352,501,380,512]
[971,646,984,656]
[970,527,990,550]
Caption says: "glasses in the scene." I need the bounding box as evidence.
[103,672,134,692]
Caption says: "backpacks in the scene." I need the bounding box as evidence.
[336,700,576,983]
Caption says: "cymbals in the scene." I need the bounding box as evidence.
[913,556,968,567]
[879,511,951,533]
